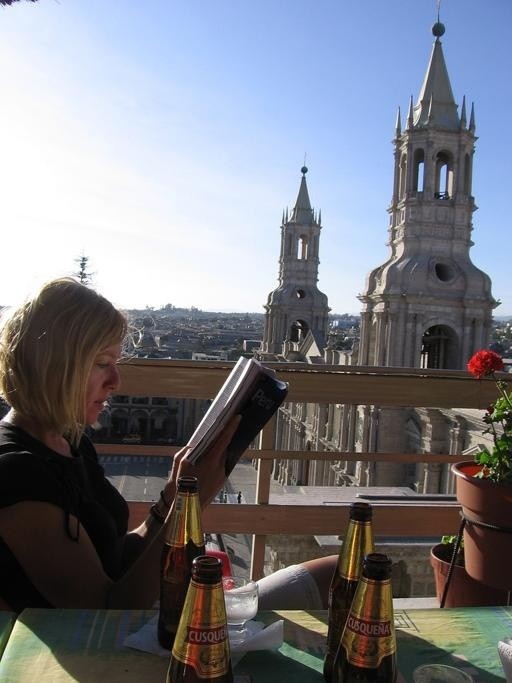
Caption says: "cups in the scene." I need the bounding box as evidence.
[412,663,474,682]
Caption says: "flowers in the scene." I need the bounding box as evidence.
[452,344,512,485]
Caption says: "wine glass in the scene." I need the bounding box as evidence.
[221,575,260,646]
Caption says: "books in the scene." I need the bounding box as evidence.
[183,352,290,476]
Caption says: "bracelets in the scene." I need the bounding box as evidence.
[148,503,166,524]
[159,489,172,510]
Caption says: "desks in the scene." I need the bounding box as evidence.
[1,594,512,683]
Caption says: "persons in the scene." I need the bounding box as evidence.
[236,490,242,502]
[219,489,224,502]
[0,275,339,614]
[223,490,227,502]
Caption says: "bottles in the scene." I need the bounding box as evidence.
[168,557,236,682]
[159,476,207,648]
[322,500,375,683]
[334,551,398,681]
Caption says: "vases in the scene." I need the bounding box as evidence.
[453,454,509,588]
[430,539,505,608]
